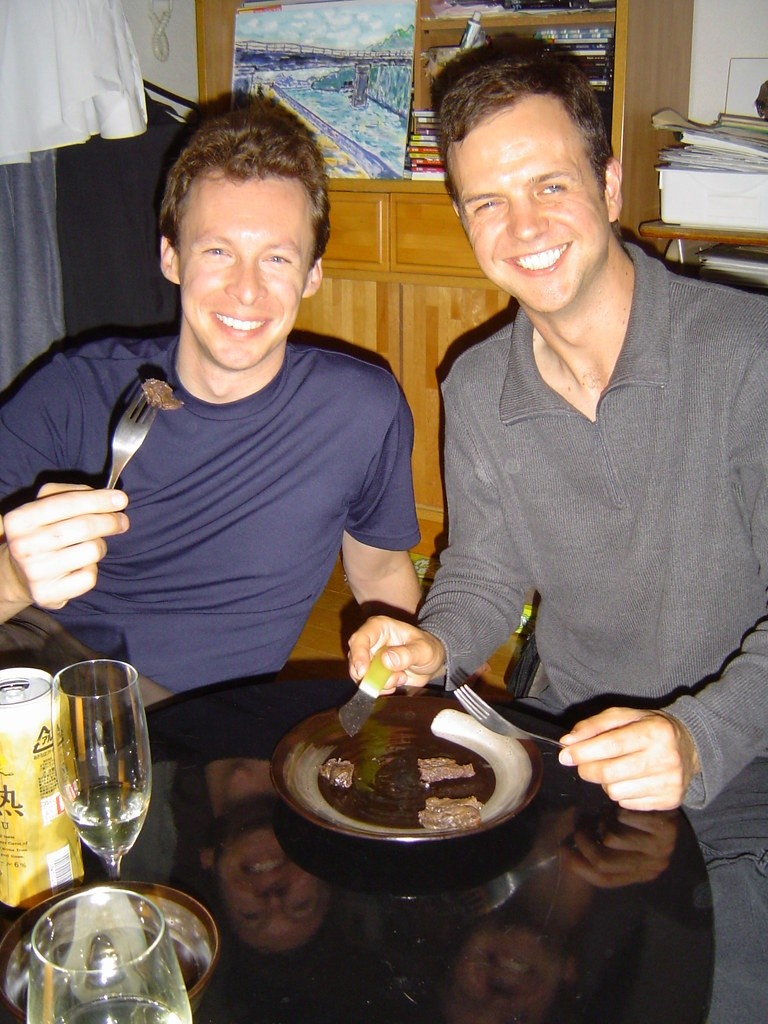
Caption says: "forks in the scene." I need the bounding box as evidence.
[269,695,542,842]
[106,387,160,489]
[453,684,565,749]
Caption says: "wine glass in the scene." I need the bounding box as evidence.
[52,659,153,881]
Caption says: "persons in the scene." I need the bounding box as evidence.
[344,57,768,1023]
[79,679,334,957]
[0,104,423,709]
[436,760,679,1023]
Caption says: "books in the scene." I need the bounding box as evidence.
[408,111,445,172]
[534,28,615,94]
[654,111,768,174]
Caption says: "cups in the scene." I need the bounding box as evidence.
[26,888,194,1024]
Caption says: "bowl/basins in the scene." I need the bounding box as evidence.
[0,881,221,1024]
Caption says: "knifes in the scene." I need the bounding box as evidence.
[338,645,394,738]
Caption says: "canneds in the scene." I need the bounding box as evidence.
[0,666,86,911]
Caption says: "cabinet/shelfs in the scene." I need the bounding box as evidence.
[192,0,694,686]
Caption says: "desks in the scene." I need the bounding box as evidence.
[637,220,768,298]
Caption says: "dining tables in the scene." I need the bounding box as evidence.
[0,673,717,1024]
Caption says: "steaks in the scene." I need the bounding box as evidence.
[319,756,354,788]
[416,796,486,832]
[142,378,184,410]
[417,757,475,783]
